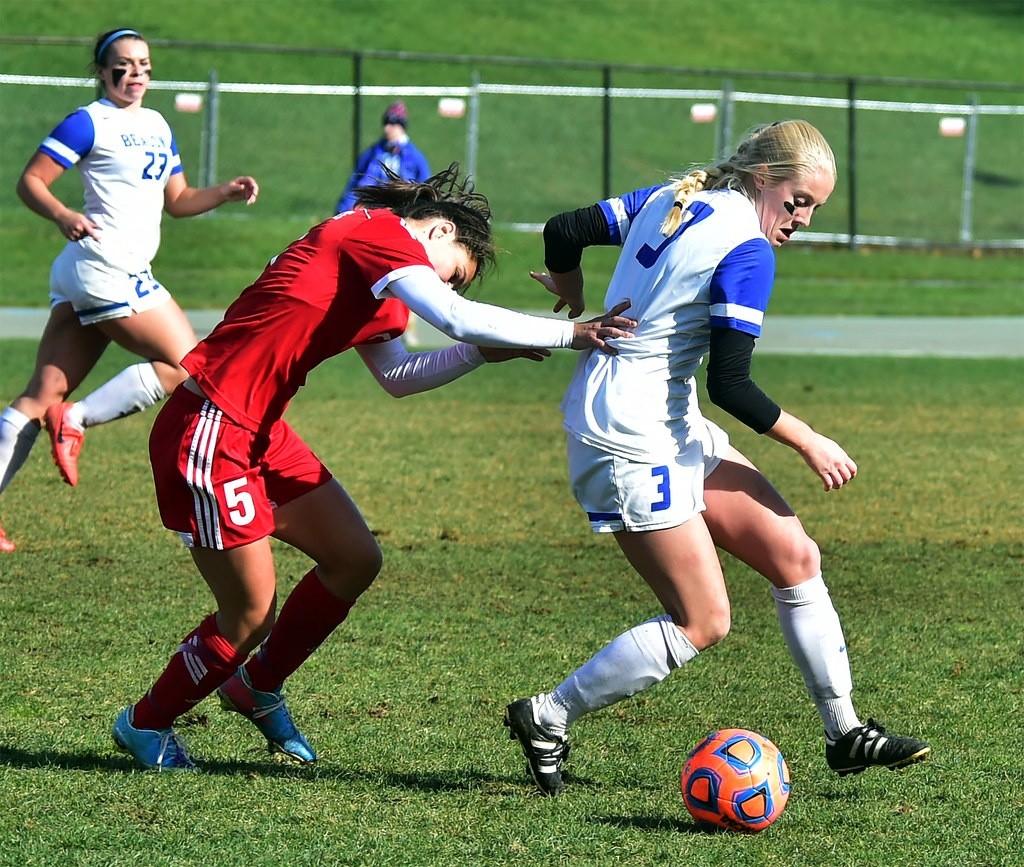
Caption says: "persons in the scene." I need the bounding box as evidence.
[335,104,430,218]
[112,165,636,774]
[502,120,929,802]
[0,29,260,550]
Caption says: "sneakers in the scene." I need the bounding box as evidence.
[503,693,576,796]
[43,402,86,488]
[0,527,18,552]
[216,664,318,765]
[824,717,930,777]
[112,705,201,774]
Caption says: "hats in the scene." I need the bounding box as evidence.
[382,102,407,128]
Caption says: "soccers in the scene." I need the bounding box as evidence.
[682,729,791,831]
